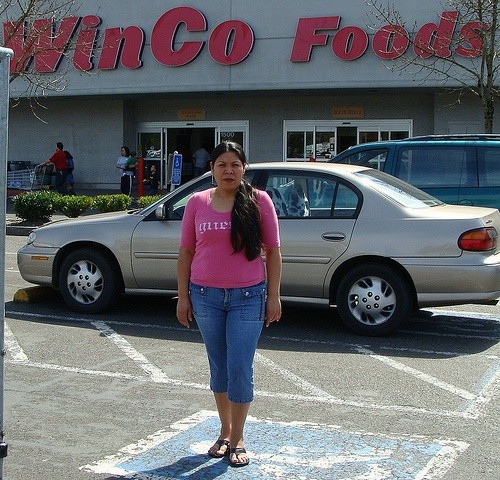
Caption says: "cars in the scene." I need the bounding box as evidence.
[16,162,500,337]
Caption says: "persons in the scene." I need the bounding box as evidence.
[37,142,76,196]
[176,141,282,467]
[116,146,160,197]
[192,144,211,176]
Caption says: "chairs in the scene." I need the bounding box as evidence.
[266,188,289,216]
[282,181,311,217]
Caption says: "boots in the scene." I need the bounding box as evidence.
[69,187,77,196]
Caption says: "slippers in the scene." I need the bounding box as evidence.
[228,446,250,467]
[208,436,230,458]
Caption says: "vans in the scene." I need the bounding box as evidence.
[270,133,500,217]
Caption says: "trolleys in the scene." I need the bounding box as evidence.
[7,160,56,191]
